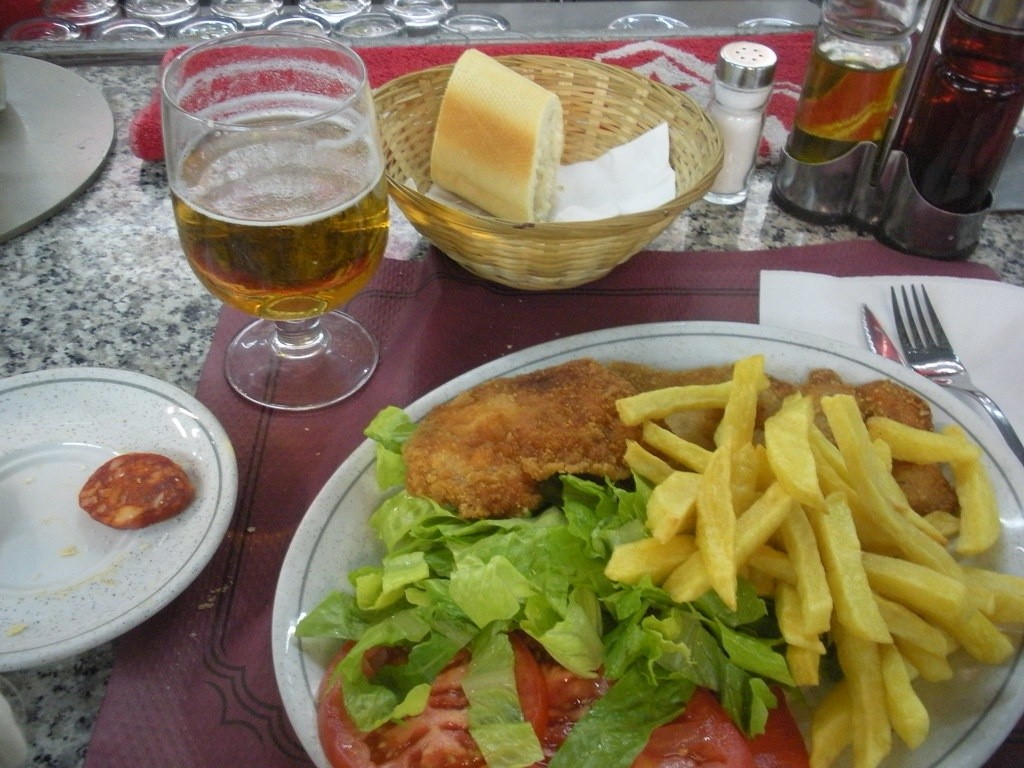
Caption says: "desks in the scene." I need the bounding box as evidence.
[0,23,1024,768]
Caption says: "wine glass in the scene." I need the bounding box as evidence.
[160,42,390,413]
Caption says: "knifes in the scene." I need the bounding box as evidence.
[859,302,915,372]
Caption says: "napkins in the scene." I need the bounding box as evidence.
[427,121,677,222]
[758,269,1024,469]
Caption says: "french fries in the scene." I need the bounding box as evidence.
[606,355,1024,768]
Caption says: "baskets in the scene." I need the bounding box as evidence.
[372,55,726,291]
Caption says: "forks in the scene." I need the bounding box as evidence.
[891,283,1024,466]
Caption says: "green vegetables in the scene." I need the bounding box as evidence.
[294,406,838,768]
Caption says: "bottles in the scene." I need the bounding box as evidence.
[894,0,1024,215]
[703,40,778,205]
[786,0,927,166]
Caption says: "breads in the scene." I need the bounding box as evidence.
[430,50,565,223]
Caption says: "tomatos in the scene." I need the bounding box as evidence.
[753,682,809,768]
[319,628,547,768]
[544,657,752,768]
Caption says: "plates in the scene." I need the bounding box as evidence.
[0,366,238,672]
[270,321,1024,768]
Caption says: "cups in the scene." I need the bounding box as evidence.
[0,0,511,72]
